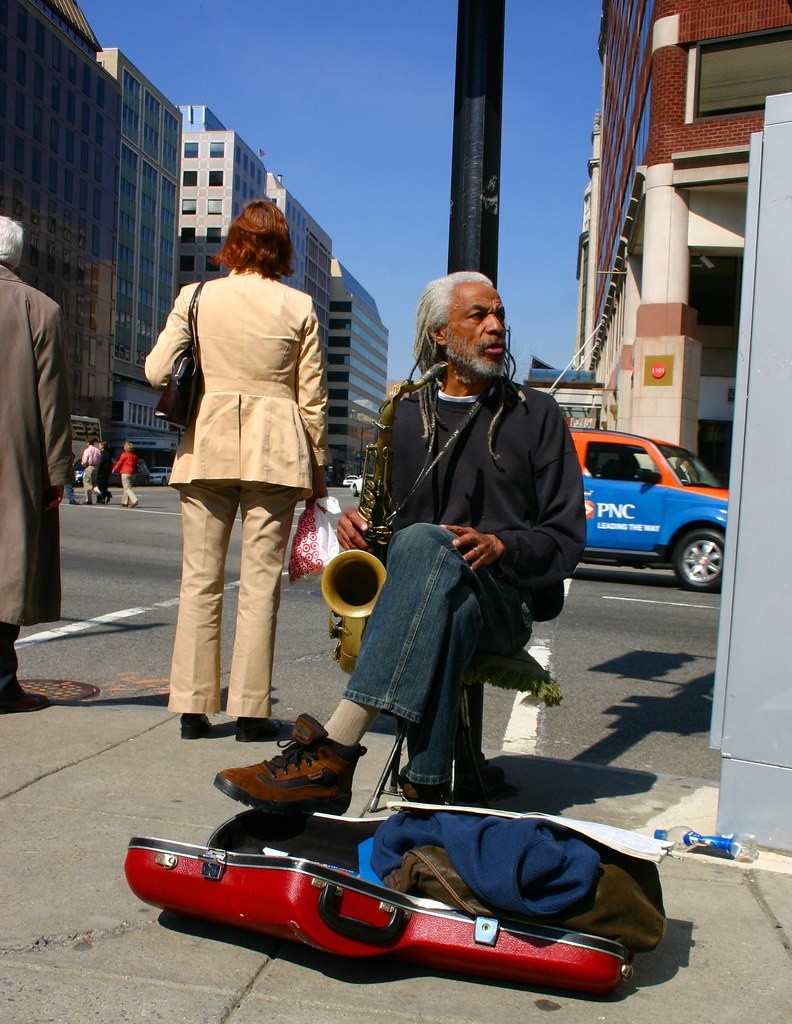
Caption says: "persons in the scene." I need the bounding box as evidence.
[144,200,333,739]
[112,443,141,508]
[212,272,587,813]
[676,459,693,483]
[65,484,80,504]
[80,437,112,504]
[0,215,76,715]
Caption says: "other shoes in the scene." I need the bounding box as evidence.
[122,503,128,507]
[131,502,139,508]
[96,493,102,503]
[86,501,92,504]
[69,500,76,504]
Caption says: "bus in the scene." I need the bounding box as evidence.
[71,414,102,465]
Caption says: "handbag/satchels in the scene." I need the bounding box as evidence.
[136,464,143,473]
[287,495,343,583]
[156,280,208,427]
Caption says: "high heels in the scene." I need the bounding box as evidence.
[101,499,105,504]
[106,494,112,503]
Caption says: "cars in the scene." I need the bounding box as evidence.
[72,457,150,486]
[569,426,729,593]
[343,475,358,486]
[326,475,341,487]
[350,474,373,497]
[149,467,172,485]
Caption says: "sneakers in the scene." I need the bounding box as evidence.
[214,711,368,814]
[393,766,454,818]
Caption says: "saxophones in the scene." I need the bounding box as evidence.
[320,361,451,671]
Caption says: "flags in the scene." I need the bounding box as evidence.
[259,149,265,157]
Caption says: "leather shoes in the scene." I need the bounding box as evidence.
[234,715,285,741]
[0,688,49,714]
[179,712,214,739]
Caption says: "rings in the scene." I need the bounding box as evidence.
[473,547,479,556]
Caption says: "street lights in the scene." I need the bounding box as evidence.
[359,414,364,475]
